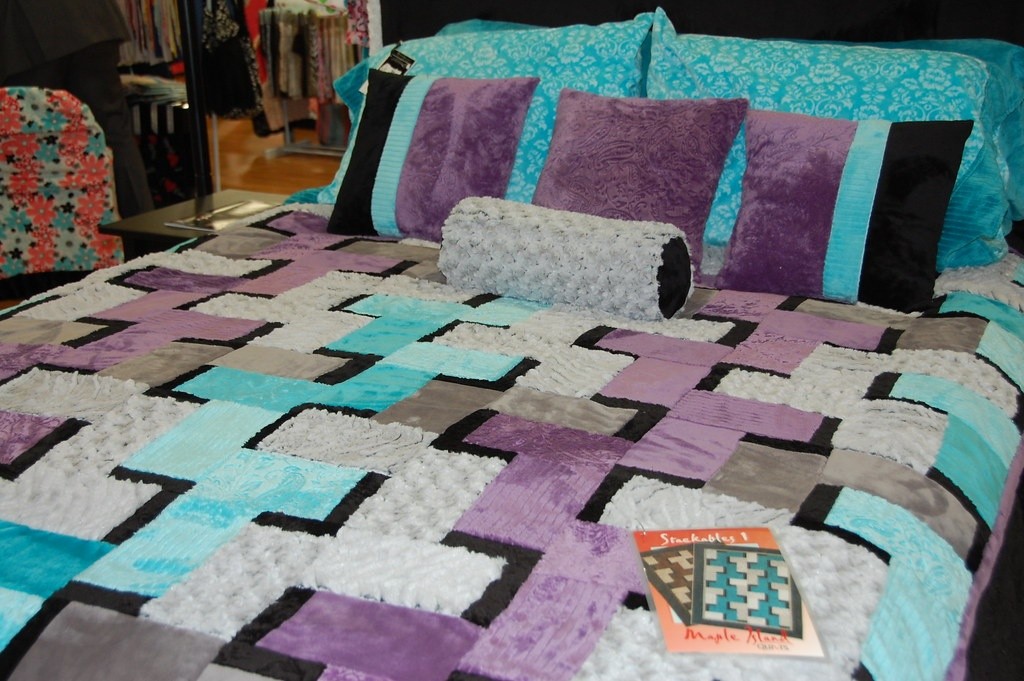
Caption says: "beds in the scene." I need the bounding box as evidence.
[0,5,1024,681]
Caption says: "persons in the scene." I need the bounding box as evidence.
[1,0,163,260]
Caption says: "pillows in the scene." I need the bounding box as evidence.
[651,6,1024,273]
[715,108,974,315]
[438,196,693,322]
[531,86,750,285]
[327,66,540,239]
[315,13,654,240]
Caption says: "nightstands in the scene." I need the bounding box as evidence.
[97,188,294,265]
